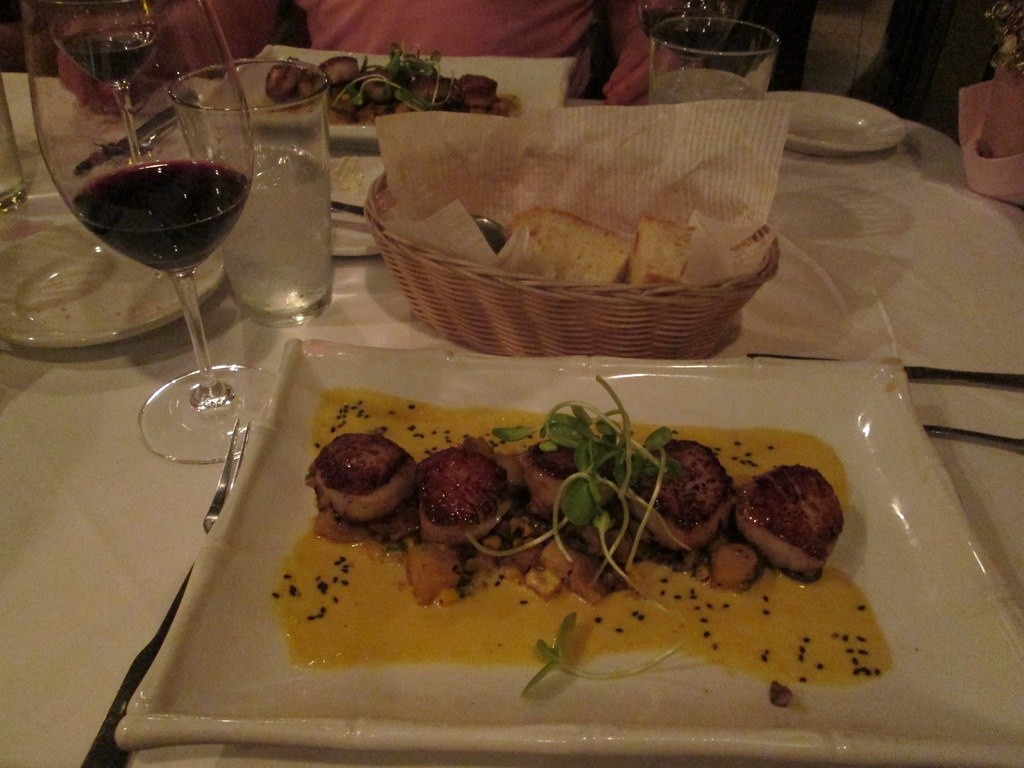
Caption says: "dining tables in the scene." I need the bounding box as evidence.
[1,46,1023,768]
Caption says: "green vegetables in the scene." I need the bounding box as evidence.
[462,374,694,695]
[312,36,458,113]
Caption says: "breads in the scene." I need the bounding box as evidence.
[503,204,695,286]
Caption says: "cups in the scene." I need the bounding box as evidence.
[0,73,27,213]
[167,59,334,326]
[647,16,781,106]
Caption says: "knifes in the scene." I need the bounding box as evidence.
[747,353,1024,393]
[74,90,198,177]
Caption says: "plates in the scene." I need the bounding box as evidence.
[207,44,578,149]
[112,337,1024,768]
[329,191,383,257]
[0,220,227,349]
[767,91,907,158]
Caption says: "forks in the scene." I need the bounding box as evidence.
[93,108,182,158]
[78,419,253,768]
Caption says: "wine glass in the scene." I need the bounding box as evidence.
[635,0,747,101]
[47,0,163,168]
[19,0,279,465]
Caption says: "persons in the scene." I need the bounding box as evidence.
[53,0,703,112]
